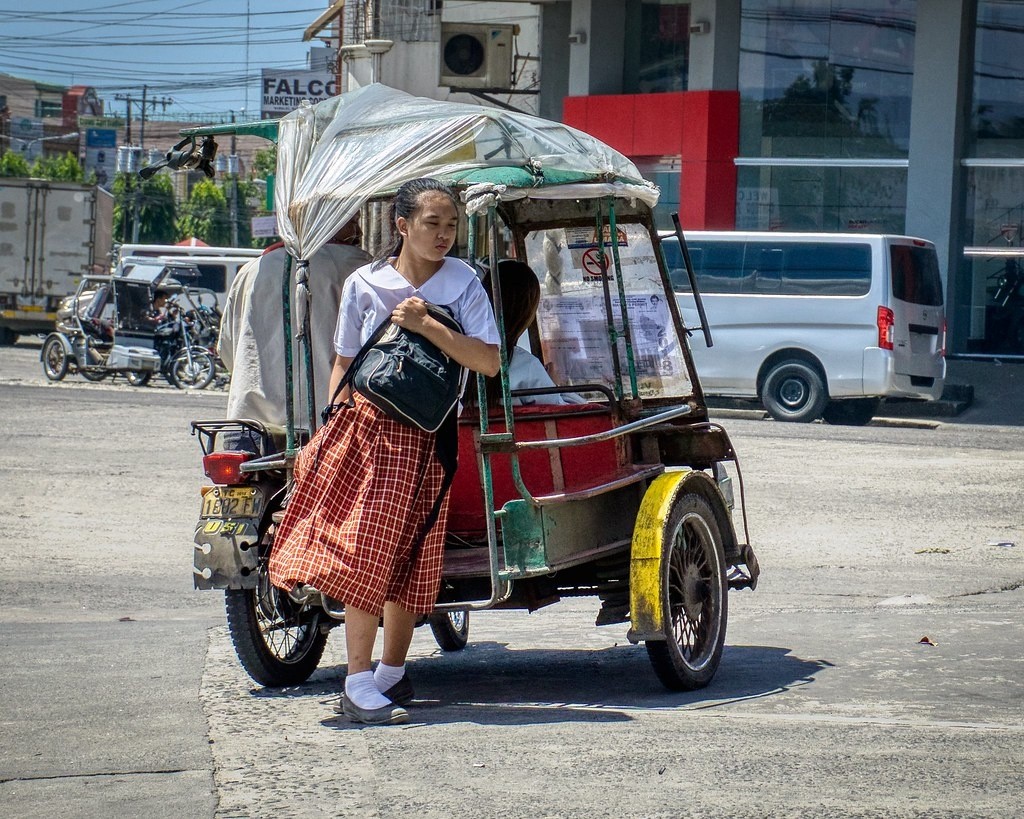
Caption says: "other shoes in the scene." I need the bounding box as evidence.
[340,671,415,725]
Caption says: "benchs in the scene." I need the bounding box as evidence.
[79,320,112,345]
[448,400,659,569]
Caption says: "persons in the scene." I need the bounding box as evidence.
[267,178,502,725]
[148,290,173,322]
[216,208,373,433]
[461,261,587,406]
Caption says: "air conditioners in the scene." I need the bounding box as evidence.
[440,20,518,89]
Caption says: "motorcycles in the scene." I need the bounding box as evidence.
[187,104,761,690]
[40,275,229,394]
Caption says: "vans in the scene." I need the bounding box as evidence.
[523,228,945,420]
[161,254,258,315]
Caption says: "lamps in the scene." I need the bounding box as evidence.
[688,22,709,34]
[568,31,586,46]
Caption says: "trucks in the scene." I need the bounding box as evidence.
[0,176,114,349]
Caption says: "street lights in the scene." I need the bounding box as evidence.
[26,132,81,160]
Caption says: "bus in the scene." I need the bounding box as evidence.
[121,244,265,259]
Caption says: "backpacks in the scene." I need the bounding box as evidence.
[321,300,470,562]
